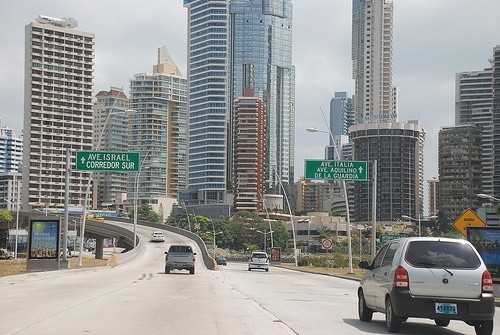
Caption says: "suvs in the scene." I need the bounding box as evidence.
[247,251,270,271]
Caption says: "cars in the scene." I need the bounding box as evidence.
[217,257,227,266]
[357,237,495,335]
[150,231,165,242]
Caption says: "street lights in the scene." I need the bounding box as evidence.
[134,150,150,248]
[206,230,223,261]
[305,105,354,274]
[265,173,298,267]
[255,230,274,252]
[401,214,439,237]
[78,85,138,267]
[177,198,191,232]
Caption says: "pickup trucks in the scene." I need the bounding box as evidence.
[164,244,197,274]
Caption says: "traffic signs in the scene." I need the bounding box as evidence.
[304,158,368,181]
